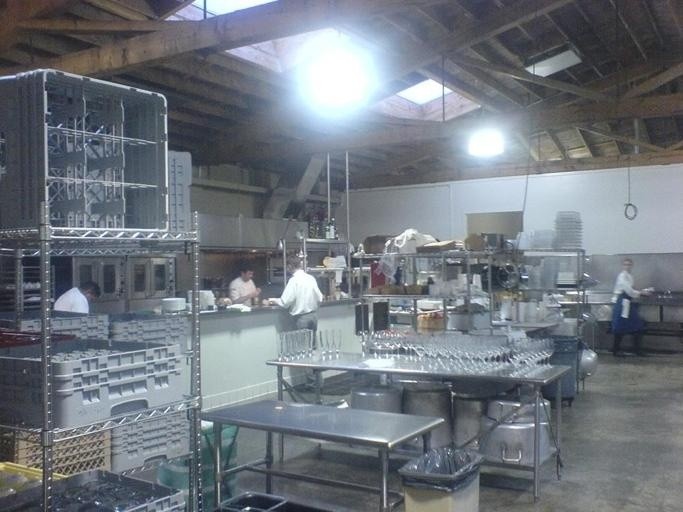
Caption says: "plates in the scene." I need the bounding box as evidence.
[189,289,215,311]
[161,297,186,311]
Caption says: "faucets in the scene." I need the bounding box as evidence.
[663,287,675,297]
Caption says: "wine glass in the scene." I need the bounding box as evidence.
[357,328,555,377]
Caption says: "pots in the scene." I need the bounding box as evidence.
[480,418,550,464]
[486,396,553,423]
[578,340,597,379]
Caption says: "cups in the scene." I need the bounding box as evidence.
[318,330,343,354]
[276,329,314,362]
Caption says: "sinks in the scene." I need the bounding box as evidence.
[658,295,673,299]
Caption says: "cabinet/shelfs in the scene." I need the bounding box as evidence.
[352,246,586,338]
[1,200,204,512]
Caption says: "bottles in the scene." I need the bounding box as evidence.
[324,216,338,240]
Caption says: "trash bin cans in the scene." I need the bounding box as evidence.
[541,335,577,407]
[397,448,485,512]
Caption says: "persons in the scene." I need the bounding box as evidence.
[49,280,101,315]
[269,256,324,393]
[225,266,262,306]
[607,257,655,360]
